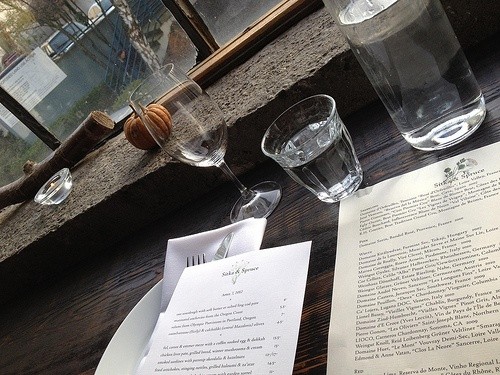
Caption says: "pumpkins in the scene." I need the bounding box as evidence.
[124,103,172,150]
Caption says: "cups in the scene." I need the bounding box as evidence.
[299,92,302,99]
[256,94,364,207]
[325,1,486,152]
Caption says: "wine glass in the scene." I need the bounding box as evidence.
[129,63,282,226]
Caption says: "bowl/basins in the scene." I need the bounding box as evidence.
[34,168,74,207]
